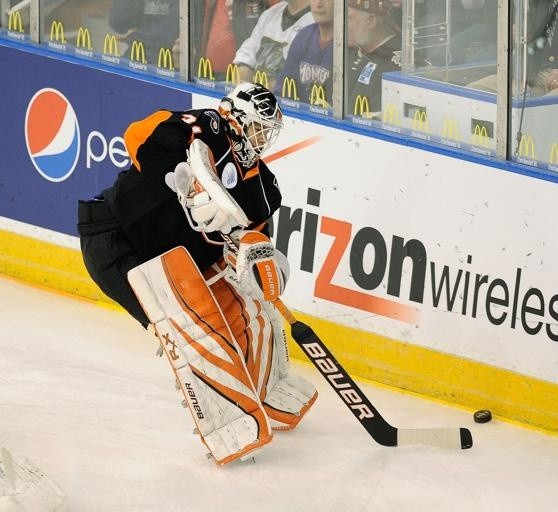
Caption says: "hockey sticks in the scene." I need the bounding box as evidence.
[219,230,474,452]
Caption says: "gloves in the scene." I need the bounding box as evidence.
[220,226,292,303]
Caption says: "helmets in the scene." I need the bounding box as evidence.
[217,79,284,169]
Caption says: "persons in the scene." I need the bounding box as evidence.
[107,1,558,124]
[76,82,320,467]
